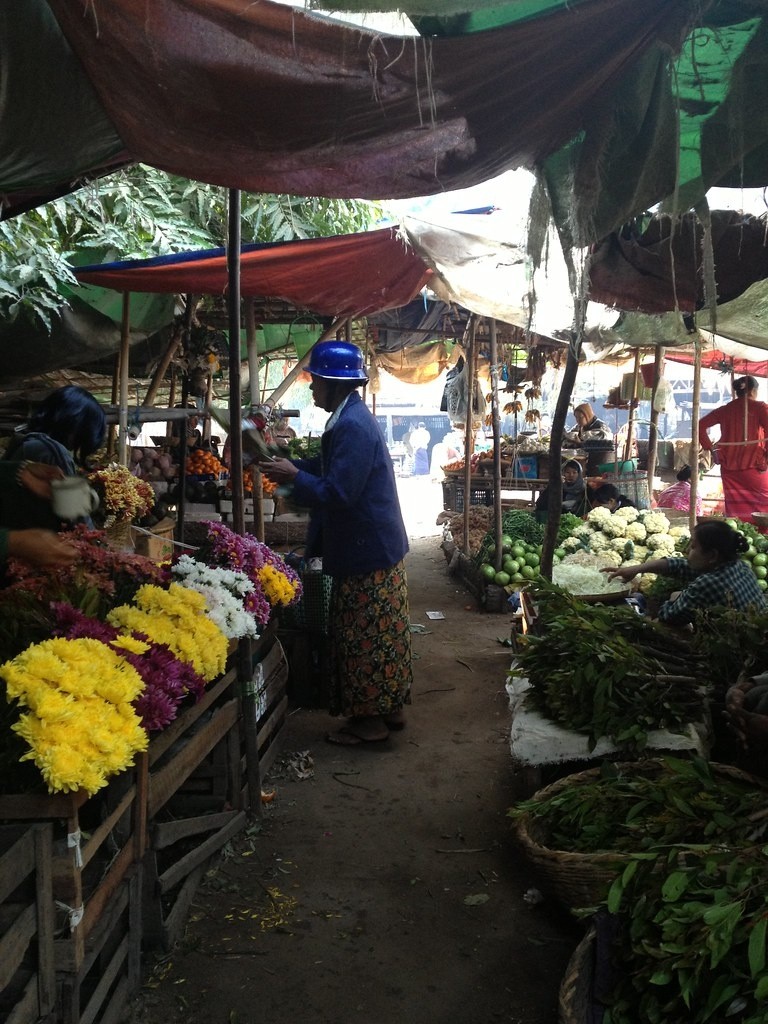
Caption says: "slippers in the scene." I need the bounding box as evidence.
[388,721,407,730]
[324,727,391,748]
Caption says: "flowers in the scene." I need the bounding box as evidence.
[0,461,305,799]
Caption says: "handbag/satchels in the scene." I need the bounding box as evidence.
[602,459,650,509]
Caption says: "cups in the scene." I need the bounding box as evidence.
[50,476,99,519]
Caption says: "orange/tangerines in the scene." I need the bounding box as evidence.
[185,449,279,493]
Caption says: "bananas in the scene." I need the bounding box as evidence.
[485,385,542,426]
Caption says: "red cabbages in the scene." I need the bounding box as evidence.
[131,449,178,480]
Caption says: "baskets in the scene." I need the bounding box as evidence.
[515,759,768,919]
[284,545,334,635]
[562,926,632,1024]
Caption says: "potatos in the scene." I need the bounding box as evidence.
[447,503,506,560]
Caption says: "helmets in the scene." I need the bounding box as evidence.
[301,341,368,382]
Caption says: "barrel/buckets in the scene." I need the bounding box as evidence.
[594,458,638,475]
[639,354,667,388]
[289,315,322,362]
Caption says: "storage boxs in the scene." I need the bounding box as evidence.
[274,511,311,523]
[219,498,275,514]
[513,455,537,479]
[135,529,174,560]
[442,480,493,512]
[183,503,217,513]
[182,512,222,522]
[139,474,180,494]
[536,455,549,480]
[226,513,273,521]
[168,470,230,490]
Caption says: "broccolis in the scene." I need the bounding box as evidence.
[556,504,690,594]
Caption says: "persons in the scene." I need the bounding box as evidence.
[535,459,595,519]
[408,421,431,476]
[656,463,702,517]
[598,519,768,637]
[256,339,415,749]
[171,402,297,466]
[560,402,613,449]
[0,384,107,534]
[0,458,83,576]
[699,375,768,534]
[593,483,636,514]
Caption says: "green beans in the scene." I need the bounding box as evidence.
[475,509,546,565]
